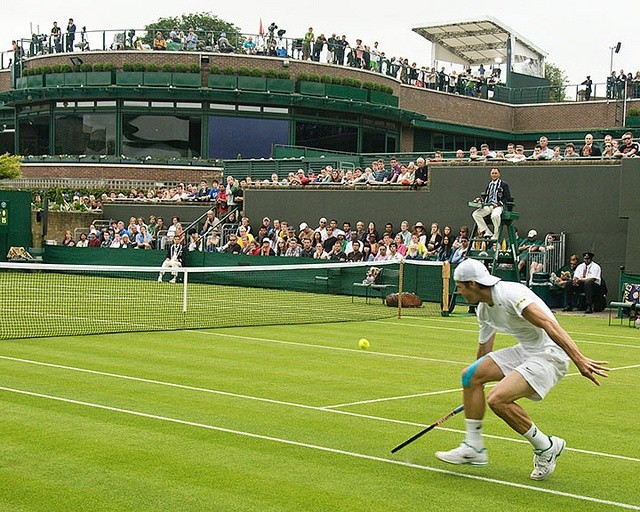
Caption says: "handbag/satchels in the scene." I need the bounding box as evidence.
[363,267,383,284]
[7,247,33,259]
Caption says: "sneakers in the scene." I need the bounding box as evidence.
[529,435,566,480]
[158,276,162,283]
[169,278,177,283]
[435,442,489,466]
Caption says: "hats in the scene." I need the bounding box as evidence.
[263,237,269,243]
[454,257,501,287]
[583,252,595,259]
[299,222,307,231]
[288,225,296,233]
[410,245,418,250]
[263,216,270,221]
[90,229,96,235]
[567,142,576,147]
[412,221,426,229]
[527,229,537,237]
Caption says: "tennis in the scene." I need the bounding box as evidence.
[358,338,369,350]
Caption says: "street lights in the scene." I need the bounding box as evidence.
[611,42,621,71]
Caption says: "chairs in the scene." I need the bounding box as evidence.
[609,283,640,329]
[370,269,400,305]
[8,246,27,262]
[314,267,342,292]
[27,247,45,262]
[353,269,381,303]
[633,303,640,328]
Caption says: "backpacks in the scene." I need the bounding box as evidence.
[386,292,420,307]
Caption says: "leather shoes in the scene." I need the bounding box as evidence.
[584,306,593,314]
[561,306,572,312]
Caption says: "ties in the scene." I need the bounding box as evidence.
[583,264,588,277]
[489,183,495,205]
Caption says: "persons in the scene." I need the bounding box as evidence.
[186,183,192,194]
[8,40,24,60]
[343,221,353,242]
[187,186,199,200]
[283,225,301,244]
[331,241,346,262]
[479,143,495,155]
[472,229,505,267]
[604,143,614,156]
[613,131,638,156]
[561,252,602,313]
[214,216,288,256]
[451,236,470,264]
[406,244,423,260]
[360,244,374,261]
[301,238,316,257]
[234,179,247,211]
[218,33,237,53]
[300,26,386,72]
[439,236,455,262]
[452,226,469,247]
[550,145,564,161]
[373,246,389,261]
[564,143,580,156]
[313,231,324,247]
[65,19,77,52]
[441,225,456,246]
[582,146,592,155]
[156,235,185,283]
[540,134,554,155]
[621,133,640,156]
[135,36,152,50]
[152,31,169,50]
[632,71,640,98]
[314,216,329,241]
[516,229,544,269]
[505,143,514,154]
[411,220,425,242]
[313,243,330,258]
[161,217,185,250]
[407,232,429,259]
[610,71,617,97]
[432,257,611,481]
[471,168,510,240]
[50,22,62,53]
[335,232,348,251]
[170,185,188,200]
[433,151,444,163]
[323,227,338,252]
[425,221,442,249]
[505,228,524,251]
[625,73,635,99]
[581,75,593,101]
[468,146,477,156]
[169,25,198,50]
[348,241,363,262]
[384,244,404,260]
[285,236,302,256]
[344,230,364,262]
[378,223,396,241]
[612,139,618,148]
[226,175,240,213]
[454,149,467,162]
[64,215,166,248]
[533,145,541,153]
[364,234,379,257]
[356,220,365,241]
[389,55,487,100]
[196,179,211,201]
[381,232,392,254]
[206,180,220,202]
[245,156,426,186]
[550,254,579,289]
[579,133,602,157]
[361,221,379,242]
[393,221,412,246]
[242,36,261,53]
[395,233,406,256]
[74,186,168,208]
[618,70,627,97]
[185,211,236,252]
[515,144,524,154]
[601,135,618,155]
[323,219,346,240]
[529,232,559,286]
[297,222,315,243]
[216,184,229,224]
[423,243,438,262]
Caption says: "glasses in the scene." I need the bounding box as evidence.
[417,226,422,228]
[625,137,631,140]
[320,221,326,224]
[380,249,384,250]
[461,240,467,242]
[264,241,269,243]
[230,239,236,241]
[570,263,576,265]
[604,145,611,147]
[459,230,467,233]
